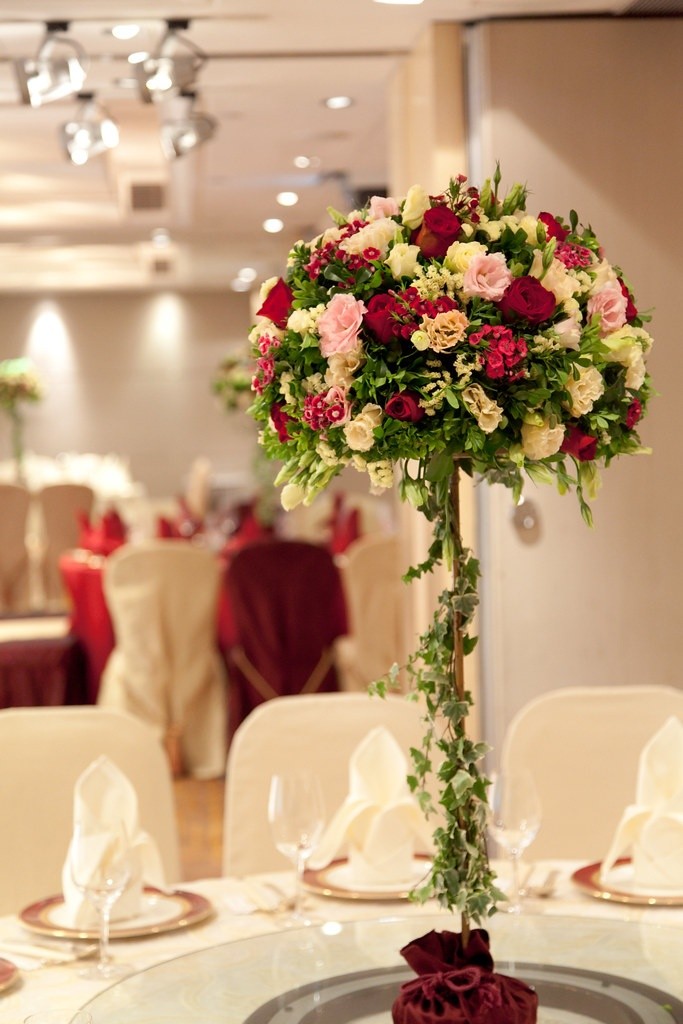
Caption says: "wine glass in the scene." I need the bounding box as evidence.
[70,815,131,977]
[267,774,326,916]
[487,770,543,912]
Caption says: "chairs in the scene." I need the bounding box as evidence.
[0,710,180,915]
[496,685,683,858]
[336,525,407,691]
[225,689,449,879]
[100,543,226,782]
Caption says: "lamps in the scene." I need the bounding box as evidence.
[58,91,119,165]
[158,90,218,159]
[18,21,86,109]
[138,18,209,104]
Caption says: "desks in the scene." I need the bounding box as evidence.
[59,543,342,708]
[0,855,683,1024]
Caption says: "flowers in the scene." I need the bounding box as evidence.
[236,162,656,1023]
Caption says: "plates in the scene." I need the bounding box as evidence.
[14,887,213,938]
[571,858,683,906]
[0,957,18,991]
[301,854,439,898]
